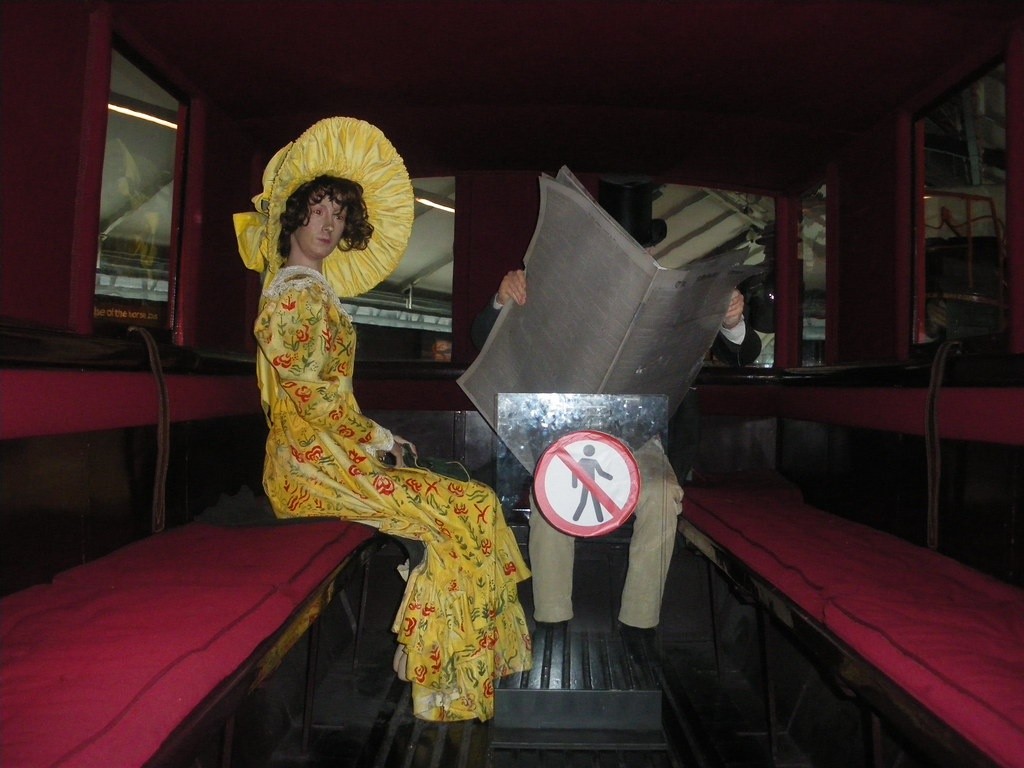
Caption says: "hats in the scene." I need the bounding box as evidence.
[598,177,667,248]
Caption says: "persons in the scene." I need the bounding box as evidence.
[231,116,532,722]
[432,339,451,360]
[473,180,764,664]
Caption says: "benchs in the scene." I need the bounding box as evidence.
[674,381,1023,768]
[2,309,497,768]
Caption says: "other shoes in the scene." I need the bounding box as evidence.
[621,624,660,667]
